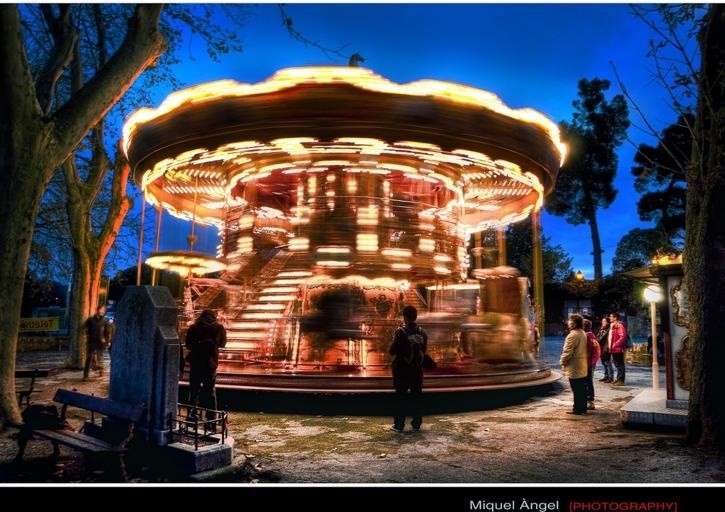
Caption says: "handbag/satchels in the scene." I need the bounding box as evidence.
[620,334,633,348]
[21,404,59,428]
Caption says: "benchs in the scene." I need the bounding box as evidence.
[3,389,148,483]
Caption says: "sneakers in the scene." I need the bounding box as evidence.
[390,424,420,432]
[565,410,580,414]
[586,401,595,409]
[598,377,625,386]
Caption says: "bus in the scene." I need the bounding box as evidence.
[32,306,72,335]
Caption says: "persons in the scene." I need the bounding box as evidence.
[605,312,628,386]
[596,317,614,384]
[559,314,588,415]
[390,305,428,433]
[582,319,601,410]
[185,308,227,431]
[83,304,108,381]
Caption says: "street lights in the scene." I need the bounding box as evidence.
[642,285,665,390]
[574,271,583,314]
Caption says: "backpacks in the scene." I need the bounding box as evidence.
[399,325,426,369]
